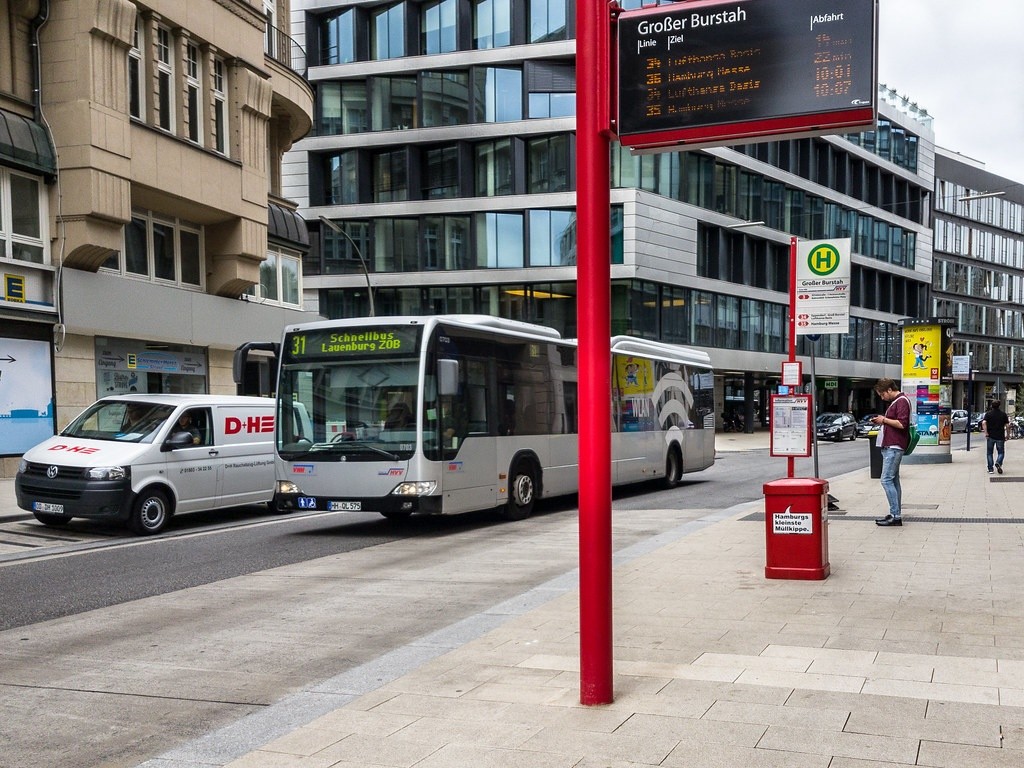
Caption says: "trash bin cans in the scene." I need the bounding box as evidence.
[763,477,831,581]
[866,430,883,478]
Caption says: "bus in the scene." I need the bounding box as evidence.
[232,313,718,524]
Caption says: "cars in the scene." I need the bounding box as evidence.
[969,412,987,433]
[855,414,884,438]
[808,411,859,442]
[949,409,969,433]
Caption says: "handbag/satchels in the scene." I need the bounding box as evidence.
[903,423,920,455]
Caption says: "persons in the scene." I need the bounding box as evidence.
[982,400,1010,474]
[168,411,201,445]
[441,399,459,438]
[871,378,911,526]
[120,402,158,435]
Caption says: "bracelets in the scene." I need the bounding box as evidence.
[882,417,886,424]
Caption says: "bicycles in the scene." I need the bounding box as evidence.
[1006,410,1024,441]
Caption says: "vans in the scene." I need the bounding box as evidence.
[15,392,314,536]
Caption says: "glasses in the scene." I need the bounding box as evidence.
[127,409,135,414]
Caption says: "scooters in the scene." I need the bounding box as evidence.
[721,409,744,433]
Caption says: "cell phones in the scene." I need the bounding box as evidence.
[870,419,875,422]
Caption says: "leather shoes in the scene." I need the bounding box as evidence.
[875,515,902,526]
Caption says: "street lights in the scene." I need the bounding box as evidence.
[319,215,375,318]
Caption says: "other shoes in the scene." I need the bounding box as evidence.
[995,462,1003,474]
[989,468,993,473]
